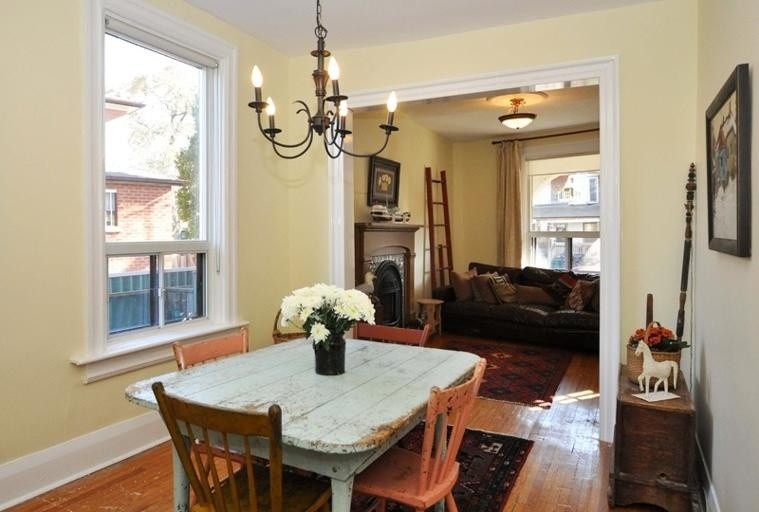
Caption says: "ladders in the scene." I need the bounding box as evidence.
[424,165,454,294]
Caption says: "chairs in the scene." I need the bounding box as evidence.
[352,321,434,348]
[146,382,286,512]
[171,327,251,512]
[347,359,487,512]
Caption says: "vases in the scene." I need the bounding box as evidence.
[312,331,346,375]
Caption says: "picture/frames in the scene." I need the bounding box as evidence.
[705,63,752,260]
[365,155,400,208]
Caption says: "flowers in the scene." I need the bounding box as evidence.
[628,327,691,352]
[280,281,378,351]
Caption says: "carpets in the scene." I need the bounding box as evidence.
[195,422,535,512]
[429,337,571,410]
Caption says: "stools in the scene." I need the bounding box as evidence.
[416,299,444,337]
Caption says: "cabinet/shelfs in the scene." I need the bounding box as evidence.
[610,361,695,512]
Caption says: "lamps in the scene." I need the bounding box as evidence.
[247,1,406,160]
[487,88,549,131]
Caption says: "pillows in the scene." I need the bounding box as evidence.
[564,279,593,313]
[514,283,556,306]
[590,278,600,313]
[448,267,479,304]
[544,271,575,304]
[469,272,498,303]
[490,273,517,304]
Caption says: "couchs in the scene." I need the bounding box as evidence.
[434,262,600,357]
